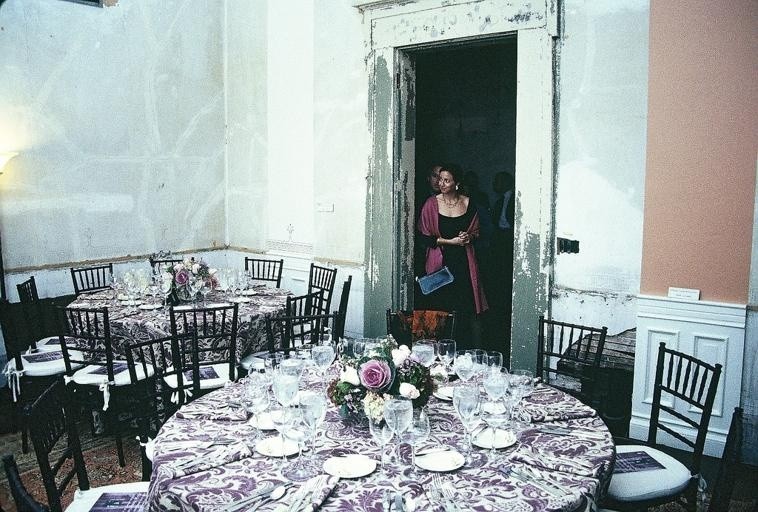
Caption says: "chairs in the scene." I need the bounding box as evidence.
[245,258,283,288]
[240,288,324,371]
[0,299,85,454]
[264,311,336,371]
[71,262,114,296]
[151,259,185,280]
[162,302,240,416]
[125,327,201,481]
[536,315,608,405]
[23,375,150,512]
[52,301,161,467]
[605,342,723,511]
[279,275,352,347]
[290,263,338,338]
[707,407,743,510]
[386,307,456,351]
[16,277,80,396]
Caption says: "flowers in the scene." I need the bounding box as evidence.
[156,259,216,302]
[327,347,432,427]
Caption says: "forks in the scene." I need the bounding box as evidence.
[288,472,340,512]
[429,472,464,512]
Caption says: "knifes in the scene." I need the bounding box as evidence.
[382,491,404,511]
[221,482,295,511]
[509,462,573,498]
[163,438,234,452]
[537,427,606,441]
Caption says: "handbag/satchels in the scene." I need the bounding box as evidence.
[418,266,454,296]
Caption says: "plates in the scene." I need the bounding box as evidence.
[470,427,517,450]
[325,453,377,477]
[235,291,257,296]
[436,387,465,403]
[256,437,305,458]
[413,449,464,473]
[248,410,294,432]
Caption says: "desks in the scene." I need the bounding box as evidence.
[144,337,615,511]
[66,278,296,433]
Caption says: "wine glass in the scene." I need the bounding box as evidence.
[368,396,430,475]
[108,266,253,316]
[353,335,397,363]
[414,338,456,381]
[453,349,532,467]
[246,342,334,468]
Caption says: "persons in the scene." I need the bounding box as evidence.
[414,162,514,351]
